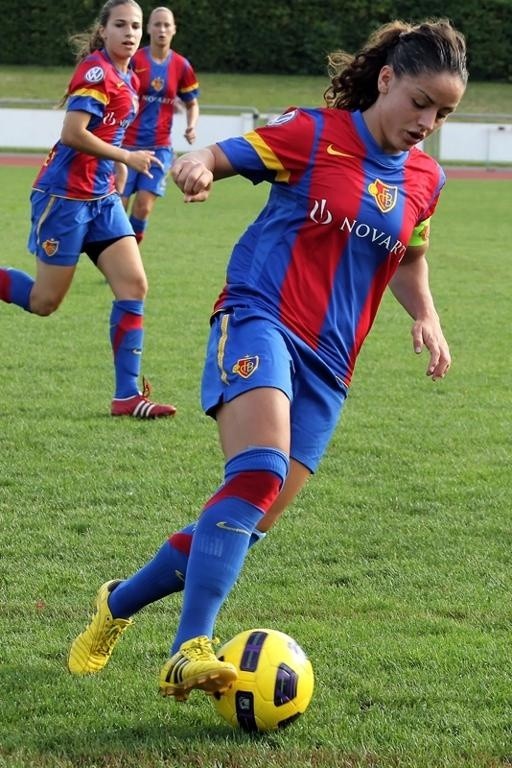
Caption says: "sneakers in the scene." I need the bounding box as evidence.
[69,579,132,676]
[160,635,238,701]
[111,377,177,418]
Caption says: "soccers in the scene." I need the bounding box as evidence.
[210,628,315,732]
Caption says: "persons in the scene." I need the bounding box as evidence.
[66,18,470,702]
[0,0,176,419]
[118,7,200,246]
